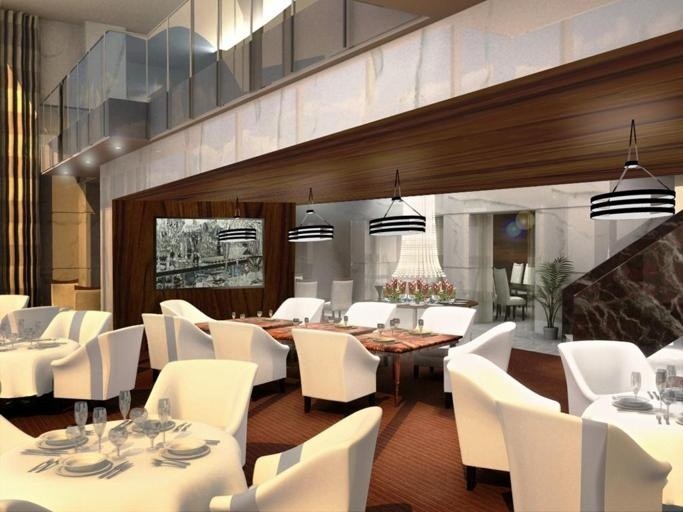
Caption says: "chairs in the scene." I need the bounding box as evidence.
[338,301,397,365]
[0,292,144,412]
[557,341,666,414]
[142,313,213,386]
[271,296,325,323]
[495,400,672,512]
[413,305,477,376]
[209,403,383,512]
[443,323,516,408]
[492,267,527,322]
[294,278,354,321]
[446,351,560,489]
[291,328,382,413]
[506,263,536,305]
[206,317,290,399]
[159,299,217,325]
[144,356,259,471]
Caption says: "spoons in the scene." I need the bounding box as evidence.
[149,454,191,470]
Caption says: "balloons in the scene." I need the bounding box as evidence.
[502,218,525,240]
[505,222,521,237]
[515,211,534,230]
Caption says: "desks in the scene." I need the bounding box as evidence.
[224,316,294,329]
[265,321,377,339]
[371,298,477,310]
[352,328,463,406]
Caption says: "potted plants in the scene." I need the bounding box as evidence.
[535,256,573,340]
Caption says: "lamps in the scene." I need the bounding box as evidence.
[283,186,336,243]
[589,119,675,220]
[217,194,256,245]
[367,171,427,235]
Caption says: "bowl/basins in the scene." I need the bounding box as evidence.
[55,452,106,472]
[39,430,67,446]
[164,439,205,454]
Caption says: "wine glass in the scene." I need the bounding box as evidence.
[73,390,176,460]
[399,292,440,304]
[0,317,42,351]
[289,315,349,330]
[231,309,272,324]
[375,317,424,337]
[612,362,682,425]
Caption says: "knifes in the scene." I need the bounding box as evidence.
[26,457,62,473]
[96,461,134,479]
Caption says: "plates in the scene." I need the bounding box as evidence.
[52,460,113,477]
[158,446,210,460]
[33,436,88,449]
